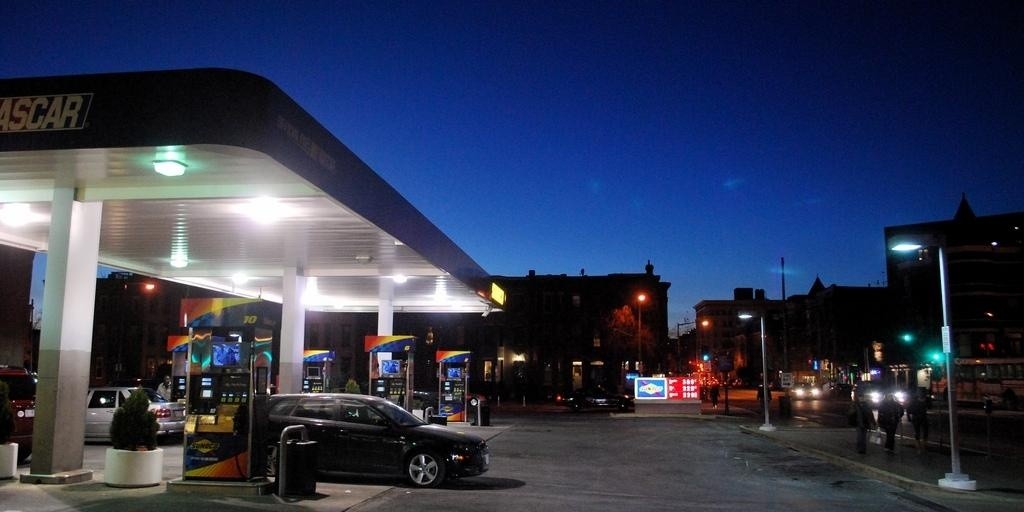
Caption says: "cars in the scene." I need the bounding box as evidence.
[264,392,490,487]
[85,386,186,441]
[851,382,908,408]
[786,382,853,400]
[552,385,635,414]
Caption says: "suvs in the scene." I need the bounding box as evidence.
[0,364,38,466]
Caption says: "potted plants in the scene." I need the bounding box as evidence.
[411,397,425,422]
[0,382,21,483]
[102,387,165,488]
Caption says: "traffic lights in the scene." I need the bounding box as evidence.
[977,343,994,351]
[900,332,913,344]
[933,353,940,361]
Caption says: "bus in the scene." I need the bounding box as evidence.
[929,356,1024,410]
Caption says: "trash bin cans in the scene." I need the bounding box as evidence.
[425,407,447,425]
[778,396,791,420]
[471,400,491,426]
[275,425,318,497]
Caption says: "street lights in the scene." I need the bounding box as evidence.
[677,319,710,372]
[736,308,777,431]
[887,232,978,492]
[637,293,646,359]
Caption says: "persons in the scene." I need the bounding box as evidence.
[907,390,931,454]
[848,389,876,454]
[157,376,171,402]
[878,393,904,451]
[712,387,719,408]
[757,384,771,409]
